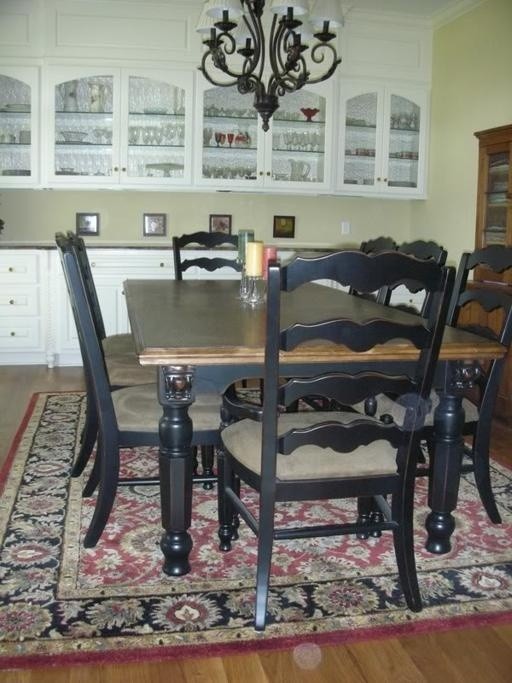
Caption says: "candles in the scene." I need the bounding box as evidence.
[264,245,277,271]
[245,240,265,277]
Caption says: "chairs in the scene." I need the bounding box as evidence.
[347,234,395,305]
[345,244,511,538]
[327,238,449,463]
[194,0,347,133]
[67,227,218,479]
[171,229,243,279]
[212,247,457,631]
[53,232,242,550]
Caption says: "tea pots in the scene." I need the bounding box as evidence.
[289,158,311,180]
[59,79,80,112]
[391,111,417,129]
[86,80,107,112]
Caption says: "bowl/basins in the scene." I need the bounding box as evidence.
[276,173,288,180]
[62,130,88,141]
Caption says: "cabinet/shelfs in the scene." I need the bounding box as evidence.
[1,60,41,188]
[455,279,512,419]
[195,64,338,195]
[51,250,240,366]
[339,79,428,198]
[0,250,51,368]
[311,278,427,313]
[41,59,194,192]
[472,122,511,279]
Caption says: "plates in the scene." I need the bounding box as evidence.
[5,169,31,175]
[4,103,32,111]
[145,108,168,114]
[61,167,73,171]
[343,179,416,187]
[486,159,510,245]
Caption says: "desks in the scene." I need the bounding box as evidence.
[121,278,510,577]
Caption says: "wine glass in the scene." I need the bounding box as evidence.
[129,122,184,147]
[204,105,326,150]
[88,125,114,143]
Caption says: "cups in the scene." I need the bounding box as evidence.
[346,147,418,159]
[203,165,257,179]
[2,130,30,144]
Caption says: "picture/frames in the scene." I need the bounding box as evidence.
[272,214,295,237]
[209,213,232,234]
[75,211,101,236]
[142,212,167,236]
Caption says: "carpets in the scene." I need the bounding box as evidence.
[1,389,510,667]
